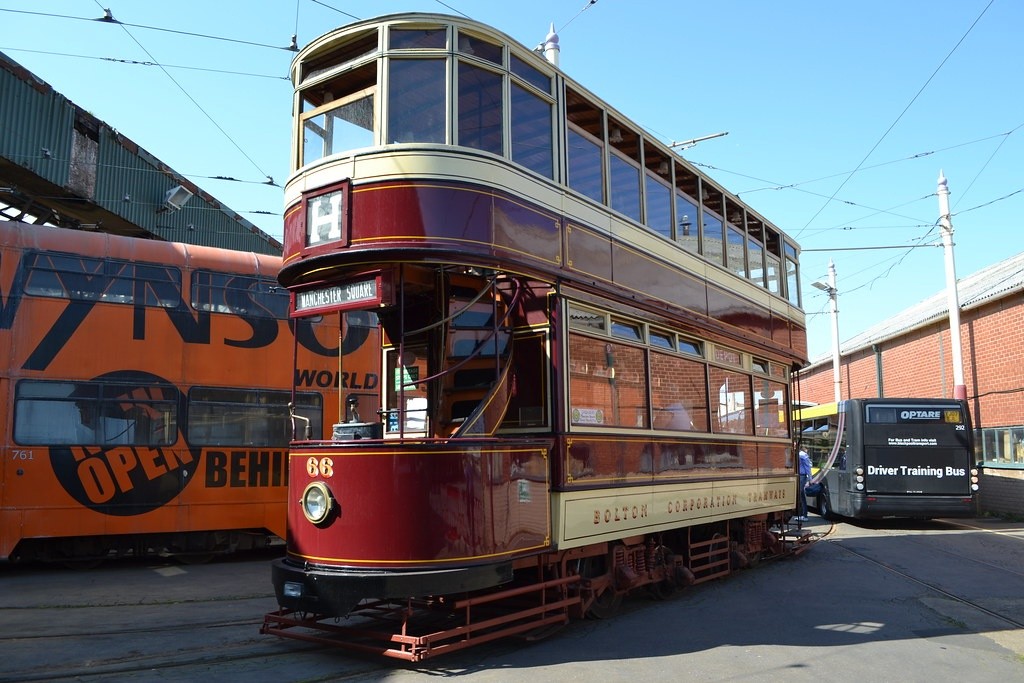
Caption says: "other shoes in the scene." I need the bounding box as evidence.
[794,516,809,522]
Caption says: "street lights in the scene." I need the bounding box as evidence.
[810,257,842,402]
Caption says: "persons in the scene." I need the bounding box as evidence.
[793,440,813,522]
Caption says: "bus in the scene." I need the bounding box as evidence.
[0,218,513,564]
[256,12,811,665]
[779,397,978,521]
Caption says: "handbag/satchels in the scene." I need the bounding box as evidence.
[805,483,821,497]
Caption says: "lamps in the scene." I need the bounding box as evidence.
[155,185,193,214]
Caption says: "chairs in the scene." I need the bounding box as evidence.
[653,408,674,429]
